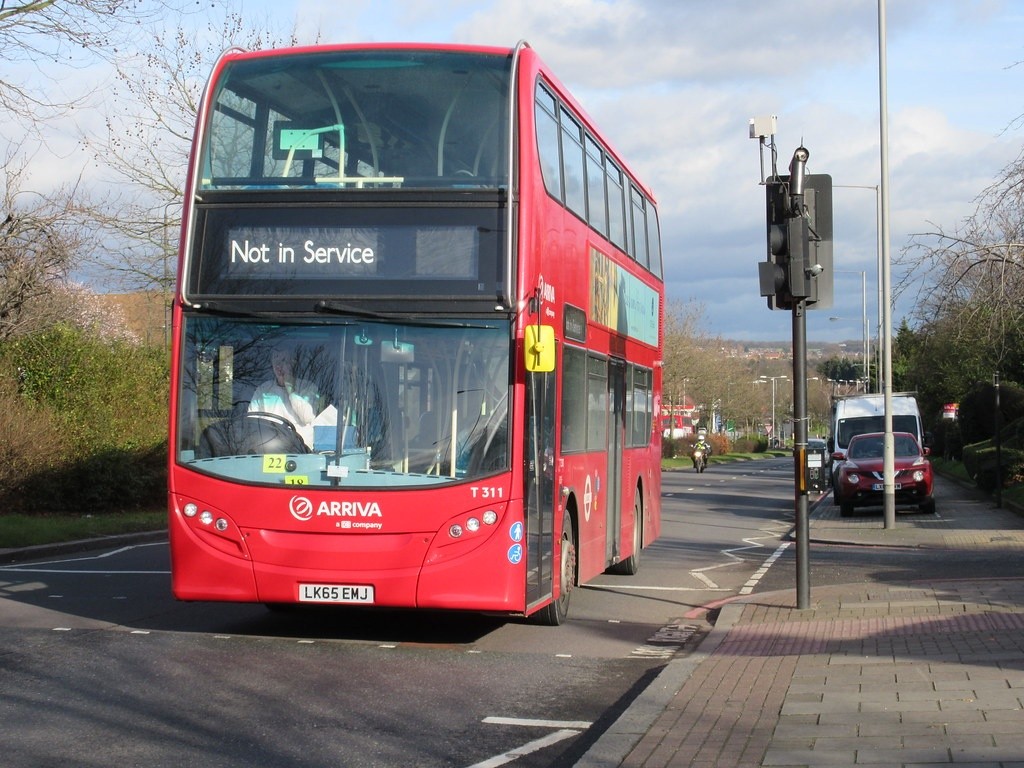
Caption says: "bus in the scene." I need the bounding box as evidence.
[169,40,662,627]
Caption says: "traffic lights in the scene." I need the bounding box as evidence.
[767,218,812,300]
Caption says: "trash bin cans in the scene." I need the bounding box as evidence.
[806,448,826,495]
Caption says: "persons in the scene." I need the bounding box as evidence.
[691,435,710,468]
[774,437,779,448]
[247,344,318,452]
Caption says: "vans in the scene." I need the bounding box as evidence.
[832,392,926,504]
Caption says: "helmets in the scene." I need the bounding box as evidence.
[698,435,705,443]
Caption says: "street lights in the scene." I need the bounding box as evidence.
[760,375,787,447]
[831,316,870,395]
[839,342,878,394]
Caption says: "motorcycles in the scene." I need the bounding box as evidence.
[690,445,710,473]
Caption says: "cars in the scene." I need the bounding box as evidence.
[831,432,936,517]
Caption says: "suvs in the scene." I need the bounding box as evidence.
[808,438,830,467]
